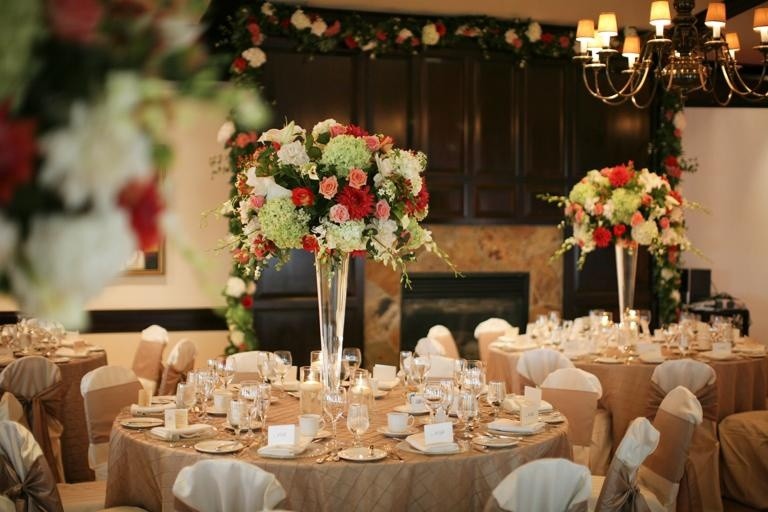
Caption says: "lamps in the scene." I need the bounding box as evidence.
[566,0,768,118]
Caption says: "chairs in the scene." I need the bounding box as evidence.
[1,306,767,511]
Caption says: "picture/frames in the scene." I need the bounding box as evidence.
[119,167,167,279]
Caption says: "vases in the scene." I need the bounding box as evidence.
[611,241,642,327]
[306,246,353,401]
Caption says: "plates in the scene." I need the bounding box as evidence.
[338,446,387,461]
[395,437,467,456]
[487,429,546,437]
[288,391,300,399]
[257,443,331,459]
[193,440,243,453]
[371,390,388,399]
[735,348,766,358]
[377,427,419,437]
[472,436,519,447]
[537,416,564,423]
[121,394,179,429]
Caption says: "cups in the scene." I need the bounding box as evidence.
[0,316,66,358]
[387,410,414,432]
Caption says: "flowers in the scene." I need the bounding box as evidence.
[214,0,274,356]
[531,156,722,275]
[272,6,573,62]
[1,0,277,340]
[656,93,700,194]
[214,116,468,294]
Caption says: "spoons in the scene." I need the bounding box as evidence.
[383,444,402,461]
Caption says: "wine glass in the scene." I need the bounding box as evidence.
[497,309,735,364]
[178,351,276,442]
[300,349,378,447]
[395,347,508,438]
[273,350,293,400]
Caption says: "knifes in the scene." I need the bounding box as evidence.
[369,444,374,456]
[316,447,342,463]
[233,440,254,456]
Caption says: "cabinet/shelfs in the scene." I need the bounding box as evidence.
[267,40,658,229]
